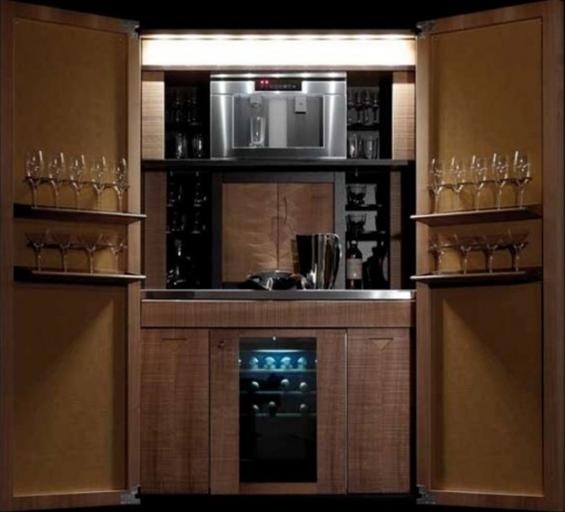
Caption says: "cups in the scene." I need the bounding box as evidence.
[295,231,343,289]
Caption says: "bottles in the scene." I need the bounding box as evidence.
[347,91,390,288]
[164,85,216,291]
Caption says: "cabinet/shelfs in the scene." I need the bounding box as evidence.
[12,197,150,287]
[345,327,411,495]
[144,326,211,493]
[211,326,346,499]
[411,202,542,288]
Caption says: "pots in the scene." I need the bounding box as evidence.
[244,270,302,291]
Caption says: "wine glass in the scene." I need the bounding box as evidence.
[22,147,128,276]
[427,148,533,275]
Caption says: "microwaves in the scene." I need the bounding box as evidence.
[211,73,346,166]
